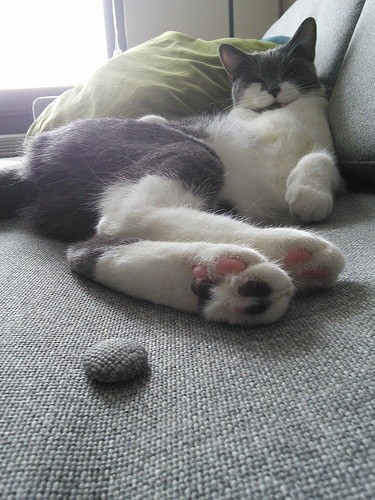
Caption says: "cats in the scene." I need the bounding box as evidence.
[0,16,346,327]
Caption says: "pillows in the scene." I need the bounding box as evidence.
[26,30,281,141]
